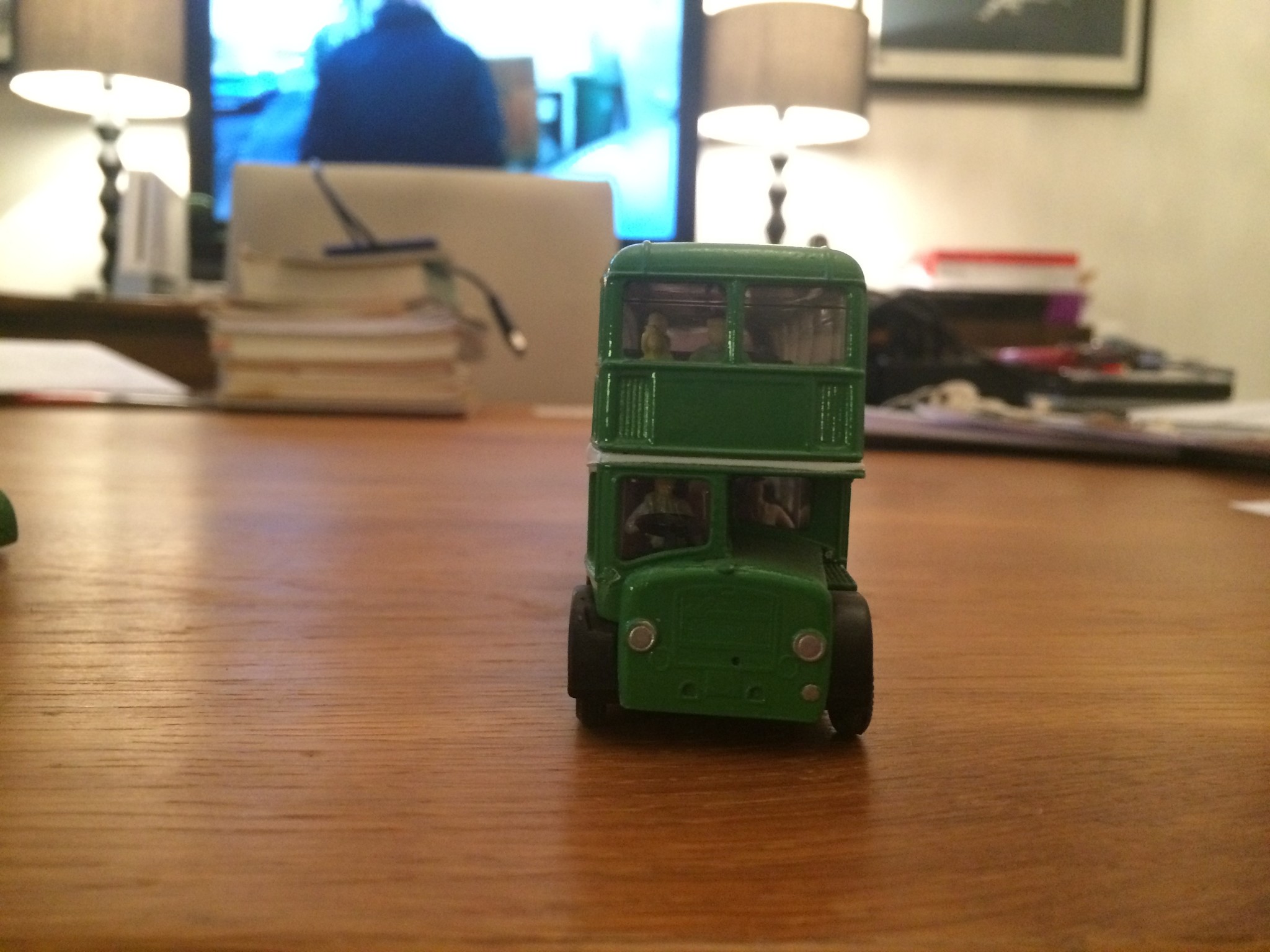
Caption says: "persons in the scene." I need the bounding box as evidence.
[301,0,512,170]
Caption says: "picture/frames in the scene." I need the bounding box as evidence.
[856,2,1152,102]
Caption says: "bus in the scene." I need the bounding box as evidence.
[567,239,879,739]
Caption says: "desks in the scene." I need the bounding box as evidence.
[0,401,1266,952]
[3,290,1071,416]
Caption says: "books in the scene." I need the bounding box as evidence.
[208,227,478,422]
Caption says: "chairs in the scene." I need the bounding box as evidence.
[218,162,613,399]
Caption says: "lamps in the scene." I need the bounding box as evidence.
[8,1,191,301]
[696,2,868,244]
